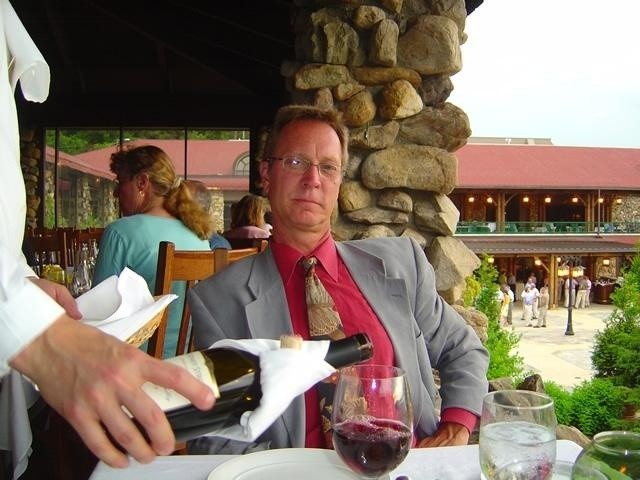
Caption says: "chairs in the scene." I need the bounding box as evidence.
[146,240,270,361]
[23,226,106,266]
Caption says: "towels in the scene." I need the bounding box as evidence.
[203,338,337,442]
[0,267,180,479]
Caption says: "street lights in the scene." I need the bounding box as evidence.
[557,254,585,335]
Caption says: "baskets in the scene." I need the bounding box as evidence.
[122,295,166,349]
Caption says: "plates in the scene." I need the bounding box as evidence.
[481,456,611,480]
[205,447,390,480]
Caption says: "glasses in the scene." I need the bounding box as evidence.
[258,152,347,179]
[113,179,120,186]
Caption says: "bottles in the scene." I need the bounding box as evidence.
[40,252,48,271]
[566,429,638,478]
[47,265,66,284]
[72,243,92,296]
[33,251,41,277]
[64,266,74,285]
[99,334,373,455]
[89,238,100,276]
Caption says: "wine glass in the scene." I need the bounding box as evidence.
[478,390,558,480]
[334,364,416,480]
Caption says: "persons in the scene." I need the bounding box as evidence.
[495,272,594,329]
[188,102,492,457]
[180,178,233,250]
[88,144,212,358]
[0,0,216,480]
[221,193,271,239]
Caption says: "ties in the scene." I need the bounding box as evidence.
[298,257,367,449]
